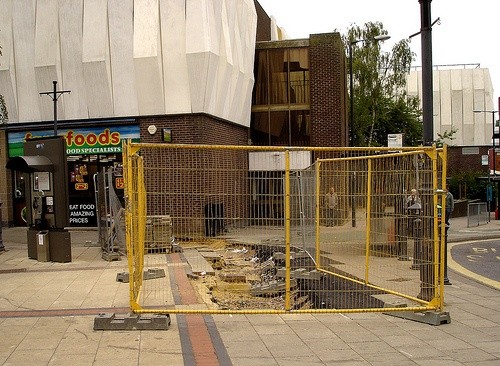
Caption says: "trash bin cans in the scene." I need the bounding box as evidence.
[36,230,49,261]
[205,202,224,235]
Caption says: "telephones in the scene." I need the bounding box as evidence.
[33,195,45,215]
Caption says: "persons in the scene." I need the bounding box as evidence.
[324,188,339,227]
[403,189,422,214]
[445,186,454,225]
